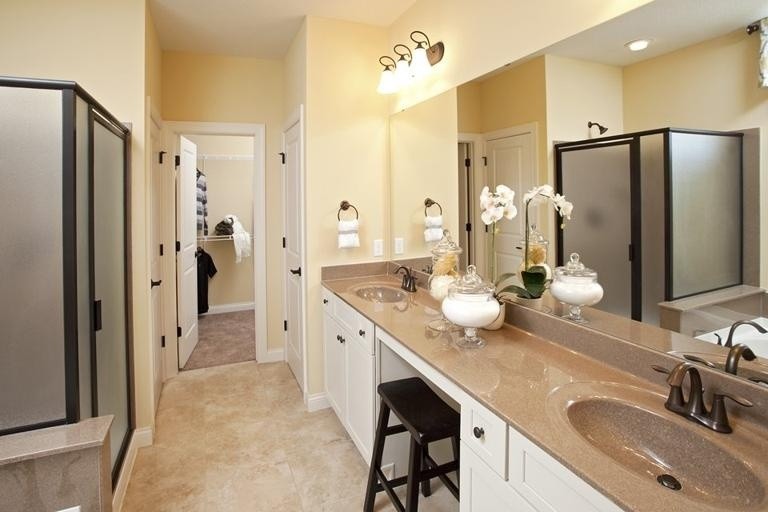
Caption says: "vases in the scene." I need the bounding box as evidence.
[482,299,506,331]
[514,293,543,312]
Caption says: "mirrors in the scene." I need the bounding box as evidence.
[456,0,768,389]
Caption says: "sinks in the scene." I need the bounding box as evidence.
[692,316,767,361]
[711,359,768,389]
[564,395,768,512]
[356,285,406,303]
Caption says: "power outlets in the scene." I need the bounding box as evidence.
[373,239,382,257]
[395,237,404,255]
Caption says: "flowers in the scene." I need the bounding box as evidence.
[479,183,532,304]
[520,184,573,299]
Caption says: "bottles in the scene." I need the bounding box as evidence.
[428,229,464,332]
[516,223,552,314]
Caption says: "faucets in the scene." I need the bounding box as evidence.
[724,318,765,347]
[650,365,754,434]
[422,264,433,274]
[721,342,757,377]
[394,266,418,292]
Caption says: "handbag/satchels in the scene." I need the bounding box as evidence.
[215,221,233,235]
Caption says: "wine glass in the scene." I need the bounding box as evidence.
[443,286,501,349]
[548,266,603,326]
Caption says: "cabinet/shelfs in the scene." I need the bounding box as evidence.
[460,391,626,512]
[323,283,376,467]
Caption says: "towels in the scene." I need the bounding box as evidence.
[338,219,360,249]
[424,216,443,242]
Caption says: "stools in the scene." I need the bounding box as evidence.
[363,376,460,512]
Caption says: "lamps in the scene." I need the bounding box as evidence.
[377,30,445,94]
[588,121,608,135]
[624,39,648,51]
